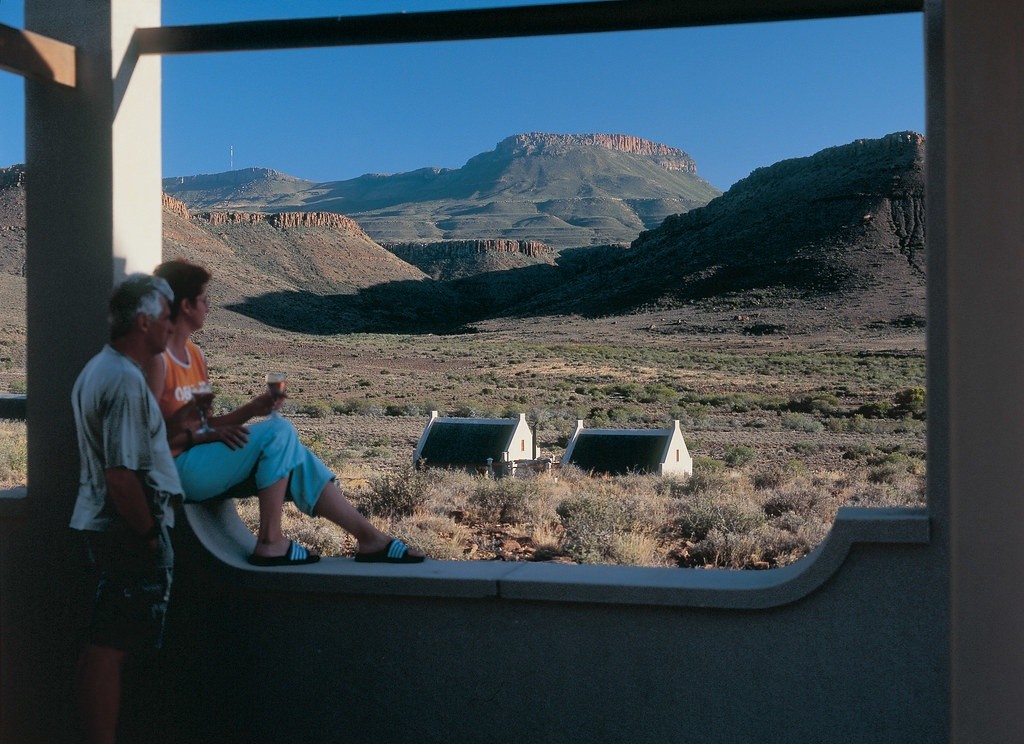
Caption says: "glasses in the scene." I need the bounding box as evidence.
[192,295,212,309]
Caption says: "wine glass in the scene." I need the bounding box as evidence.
[189,382,214,433]
[266,373,285,419]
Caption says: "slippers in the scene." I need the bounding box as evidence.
[355,535,426,563]
[247,539,320,566]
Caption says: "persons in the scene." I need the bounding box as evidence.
[137,259,427,567]
[66,271,216,744]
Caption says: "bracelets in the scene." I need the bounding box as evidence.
[182,428,194,451]
[142,526,158,542]
